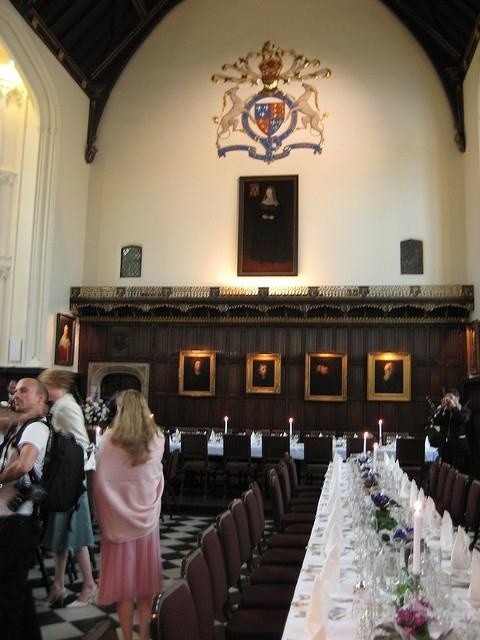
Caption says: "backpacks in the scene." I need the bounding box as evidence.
[13,417,85,512]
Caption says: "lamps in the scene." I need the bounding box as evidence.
[401,240,423,274]
[120,246,142,277]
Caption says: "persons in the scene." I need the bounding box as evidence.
[36,365,99,608]
[192,359,204,375]
[316,363,330,375]
[0,376,58,640]
[250,184,292,263]
[258,364,268,375]
[426,388,478,480]
[92,389,168,639]
[57,322,74,362]
[383,362,396,380]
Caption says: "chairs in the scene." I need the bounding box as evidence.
[35,512,97,595]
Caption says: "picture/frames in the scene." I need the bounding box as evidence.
[367,352,411,401]
[246,353,281,394]
[466,319,480,375]
[237,175,298,276]
[305,352,347,401]
[55,313,76,366]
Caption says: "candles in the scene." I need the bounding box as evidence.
[224,416,228,434]
[289,417,294,439]
[378,419,384,438]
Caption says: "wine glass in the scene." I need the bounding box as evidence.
[348,451,477,639]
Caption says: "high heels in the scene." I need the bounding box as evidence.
[38,587,67,608]
[66,584,98,607]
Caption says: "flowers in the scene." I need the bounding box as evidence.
[82,396,109,443]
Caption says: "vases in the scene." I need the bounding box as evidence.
[178,350,216,397]
